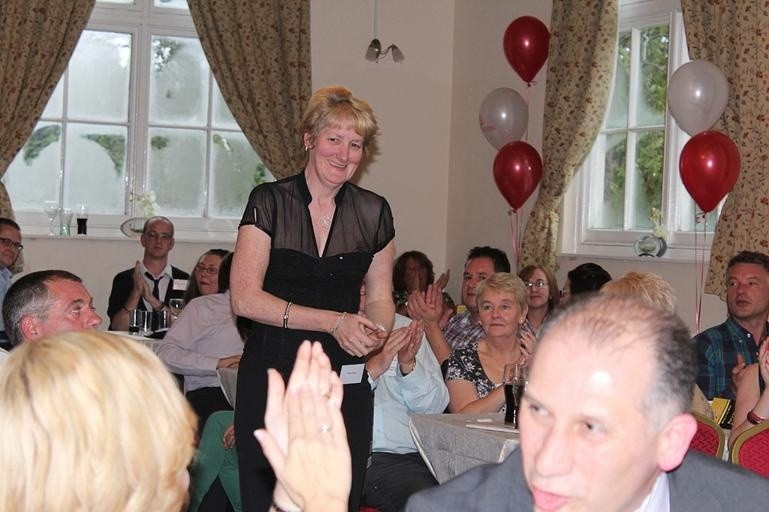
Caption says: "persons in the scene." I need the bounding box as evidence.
[1,215,246,512]
[405,291,769,511]
[601,251,769,451]
[231,86,395,512]
[360,247,612,512]
[1,330,351,512]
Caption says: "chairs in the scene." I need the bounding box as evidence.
[688,408,725,459]
[729,422,769,481]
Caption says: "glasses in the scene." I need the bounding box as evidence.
[1,236,24,252]
[524,282,546,289]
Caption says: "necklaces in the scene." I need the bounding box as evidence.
[320,216,330,225]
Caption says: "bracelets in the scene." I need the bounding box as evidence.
[330,312,346,335]
[271,502,286,512]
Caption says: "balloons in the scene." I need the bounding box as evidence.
[679,132,739,213]
[493,141,542,213]
[478,89,528,149]
[666,60,730,136]
[503,16,551,88]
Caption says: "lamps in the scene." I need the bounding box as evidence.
[365,38,406,65]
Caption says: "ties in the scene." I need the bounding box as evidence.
[146,273,164,300]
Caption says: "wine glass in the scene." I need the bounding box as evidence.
[43,200,59,236]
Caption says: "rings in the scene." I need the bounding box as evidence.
[320,428,331,433]
[322,392,330,398]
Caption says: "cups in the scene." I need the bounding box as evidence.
[501,363,529,429]
[76,204,89,236]
[128,298,185,336]
[57,208,74,234]
